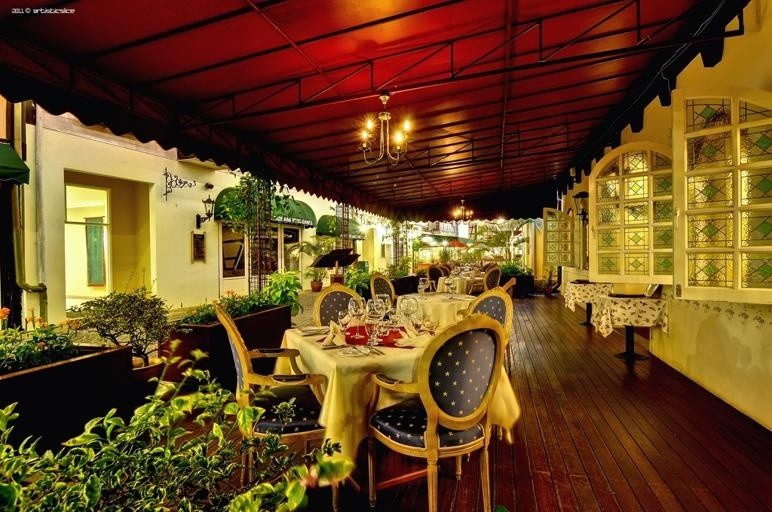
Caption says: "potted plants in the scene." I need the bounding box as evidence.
[66,290,175,402]
[287,234,342,292]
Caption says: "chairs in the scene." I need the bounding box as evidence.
[314,283,362,326]
[214,304,337,512]
[416,266,520,294]
[367,313,506,512]
[370,271,397,305]
[464,286,514,345]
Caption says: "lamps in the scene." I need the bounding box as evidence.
[196,195,217,229]
[352,90,414,168]
[572,190,590,228]
[448,199,475,225]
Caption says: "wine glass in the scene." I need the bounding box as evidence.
[418,277,430,300]
[338,294,439,346]
[444,276,457,300]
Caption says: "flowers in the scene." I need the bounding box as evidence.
[0,306,79,377]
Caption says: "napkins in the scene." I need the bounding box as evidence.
[392,324,432,348]
[323,321,348,348]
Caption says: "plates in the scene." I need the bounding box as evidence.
[336,346,370,357]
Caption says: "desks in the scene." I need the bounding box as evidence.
[499,275,535,300]
[565,281,665,361]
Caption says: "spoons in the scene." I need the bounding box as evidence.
[302,329,330,336]
